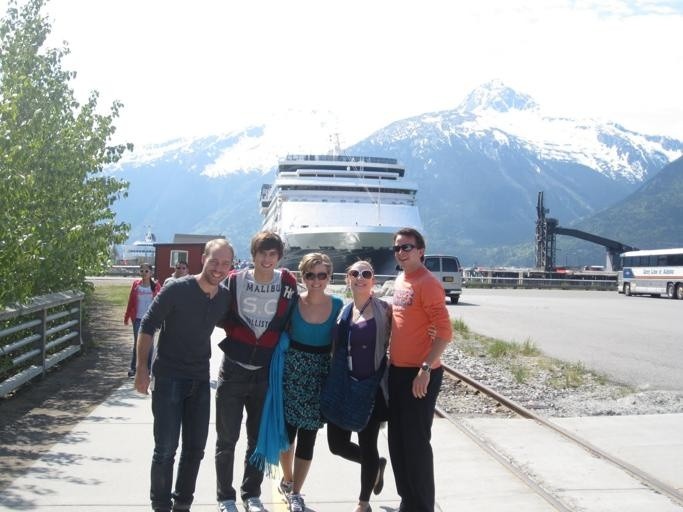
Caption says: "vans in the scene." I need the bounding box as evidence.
[418,253,465,304]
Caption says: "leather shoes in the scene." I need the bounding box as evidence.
[373,456,386,494]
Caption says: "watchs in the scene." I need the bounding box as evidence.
[420,361,430,371]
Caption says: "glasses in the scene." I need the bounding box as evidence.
[304,272,327,280]
[349,270,372,279]
[393,244,417,252]
[140,267,191,273]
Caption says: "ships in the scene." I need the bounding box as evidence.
[253,132,429,286]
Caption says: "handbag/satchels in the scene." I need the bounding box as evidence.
[320,351,388,433]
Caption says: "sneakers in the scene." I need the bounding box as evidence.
[243,495,265,512]
[128,367,137,379]
[280,476,305,512]
[220,499,237,512]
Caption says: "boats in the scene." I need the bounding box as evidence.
[120,223,162,263]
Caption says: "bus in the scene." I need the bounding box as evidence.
[614,248,683,299]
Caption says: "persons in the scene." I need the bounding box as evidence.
[276,253,342,511]
[134,238,234,512]
[216,229,297,511]
[163,261,190,284]
[122,262,161,376]
[387,227,452,512]
[321,260,437,511]
[226,257,253,278]
